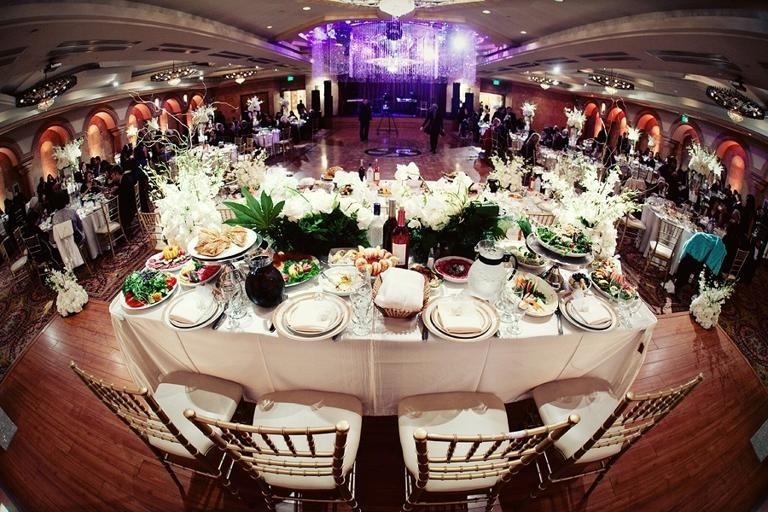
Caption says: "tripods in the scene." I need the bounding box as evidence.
[375,112,401,136]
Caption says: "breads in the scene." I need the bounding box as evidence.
[356,248,399,276]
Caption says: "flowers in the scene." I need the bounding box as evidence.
[245,95,264,113]
[519,101,537,123]
[188,104,217,128]
[563,104,588,137]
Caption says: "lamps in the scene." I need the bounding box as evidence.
[326,0,486,19]
[704,80,764,124]
[584,66,634,95]
[526,70,572,91]
[223,66,256,86]
[14,61,77,113]
[149,58,200,87]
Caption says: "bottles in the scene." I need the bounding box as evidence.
[383,197,398,251]
[240,253,285,312]
[357,157,381,186]
[392,206,409,268]
[366,202,385,246]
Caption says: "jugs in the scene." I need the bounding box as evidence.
[465,239,519,302]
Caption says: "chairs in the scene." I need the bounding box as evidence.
[2,114,751,510]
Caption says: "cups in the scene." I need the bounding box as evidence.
[650,193,727,240]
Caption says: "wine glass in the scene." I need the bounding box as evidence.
[216,269,249,328]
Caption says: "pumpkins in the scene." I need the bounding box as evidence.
[164,245,179,260]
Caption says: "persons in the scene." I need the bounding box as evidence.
[672,163,768,285]
[357,98,372,141]
[419,104,443,154]
[24,100,310,261]
[451,95,515,151]
[540,126,676,192]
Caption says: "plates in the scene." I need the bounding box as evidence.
[273,250,318,287]
[510,272,559,320]
[522,232,599,271]
[531,225,597,256]
[185,227,262,260]
[318,264,361,300]
[353,246,391,280]
[555,291,618,336]
[320,173,333,180]
[174,262,222,285]
[143,248,190,271]
[163,290,224,333]
[588,264,635,302]
[421,295,501,343]
[537,203,554,212]
[512,251,547,270]
[433,254,469,285]
[270,292,352,345]
[119,270,175,310]
